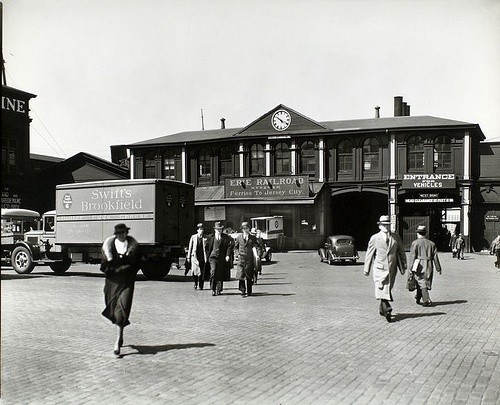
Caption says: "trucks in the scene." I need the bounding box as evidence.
[10,178,196,279]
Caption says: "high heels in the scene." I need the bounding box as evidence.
[113,350,120,354]
[119,340,123,346]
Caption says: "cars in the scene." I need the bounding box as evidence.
[318,235,359,265]
[228,233,271,264]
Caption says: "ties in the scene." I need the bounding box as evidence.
[386,234,389,246]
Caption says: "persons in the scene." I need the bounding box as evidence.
[235,222,256,297]
[491,234,500,269]
[449,234,458,258]
[187,223,209,290]
[206,222,234,296]
[101,224,141,354]
[256,230,267,276]
[408,225,441,306]
[249,227,258,286]
[364,214,407,317]
[455,233,465,260]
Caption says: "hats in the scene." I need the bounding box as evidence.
[251,228,258,234]
[240,222,252,229]
[197,223,205,228]
[415,225,428,233]
[114,224,130,234]
[376,215,391,225]
[213,222,224,229]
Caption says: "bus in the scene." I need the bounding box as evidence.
[1,208,40,263]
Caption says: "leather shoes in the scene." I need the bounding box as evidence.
[217,291,220,295]
[386,311,391,321]
[246,293,251,296]
[199,287,203,290]
[212,291,216,295]
[193,285,197,290]
[416,297,420,304]
[423,300,431,306]
[379,310,384,315]
[242,292,245,295]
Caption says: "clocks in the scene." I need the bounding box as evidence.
[271,109,292,131]
